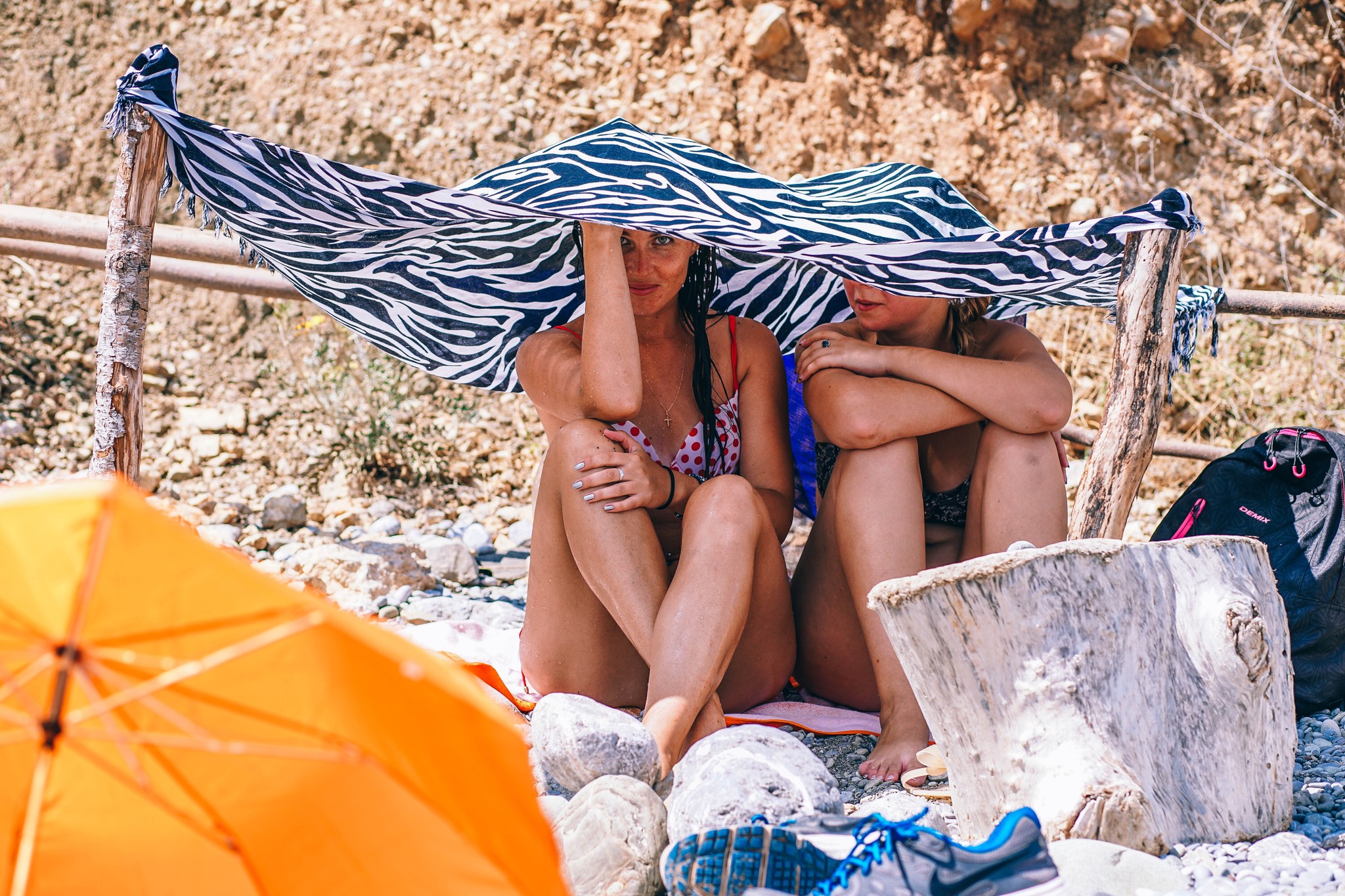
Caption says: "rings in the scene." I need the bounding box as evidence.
[822,339,830,348]
[618,468,624,481]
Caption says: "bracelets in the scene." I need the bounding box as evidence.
[656,465,675,510]
[674,473,707,520]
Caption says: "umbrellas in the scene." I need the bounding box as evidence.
[0,468,575,896]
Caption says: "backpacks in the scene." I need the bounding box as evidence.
[1148,425,1345,724]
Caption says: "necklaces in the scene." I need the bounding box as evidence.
[641,331,688,427]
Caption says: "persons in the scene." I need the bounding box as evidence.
[790,275,1073,786]
[516,221,798,786]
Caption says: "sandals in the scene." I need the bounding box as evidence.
[900,742,952,805]
